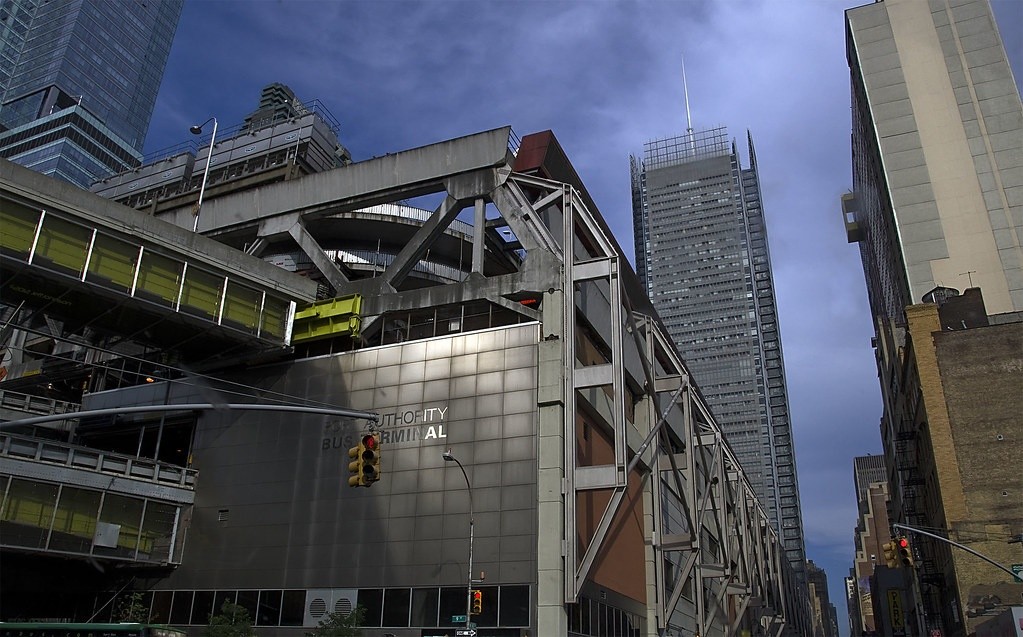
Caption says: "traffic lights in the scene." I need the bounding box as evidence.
[474,590,482,613]
[349,442,363,488]
[360,433,380,484]
[883,541,901,569]
[896,537,915,568]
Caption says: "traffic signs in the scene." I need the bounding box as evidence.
[455,629,477,637]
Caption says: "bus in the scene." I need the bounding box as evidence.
[0,622,188,637]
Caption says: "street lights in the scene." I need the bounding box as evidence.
[189,114,219,232]
[441,453,474,629]
